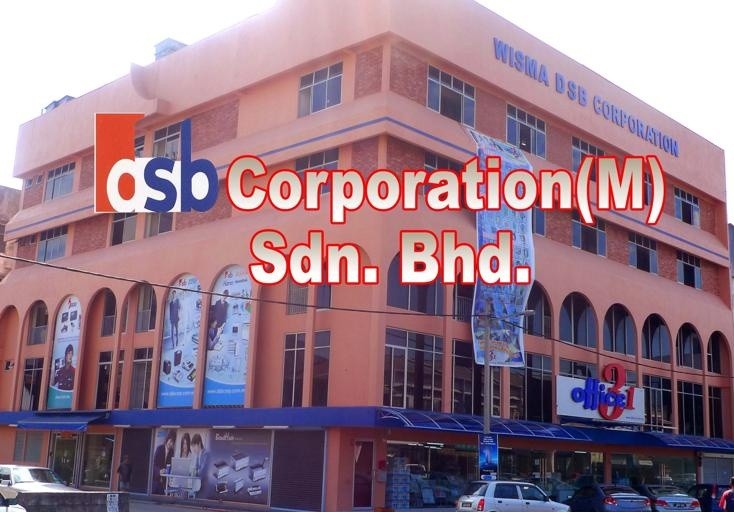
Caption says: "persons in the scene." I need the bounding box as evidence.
[49,343,75,391]
[179,432,192,458]
[191,433,210,478]
[215,290,229,328]
[719,478,734,511]
[206,306,226,350]
[169,290,181,349]
[116,453,132,490]
[152,431,176,494]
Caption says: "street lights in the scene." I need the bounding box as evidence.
[482,296,535,480]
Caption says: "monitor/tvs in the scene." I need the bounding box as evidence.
[171,458,191,476]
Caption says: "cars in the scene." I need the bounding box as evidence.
[581,482,732,512]
[458,481,572,512]
[0,463,80,506]
